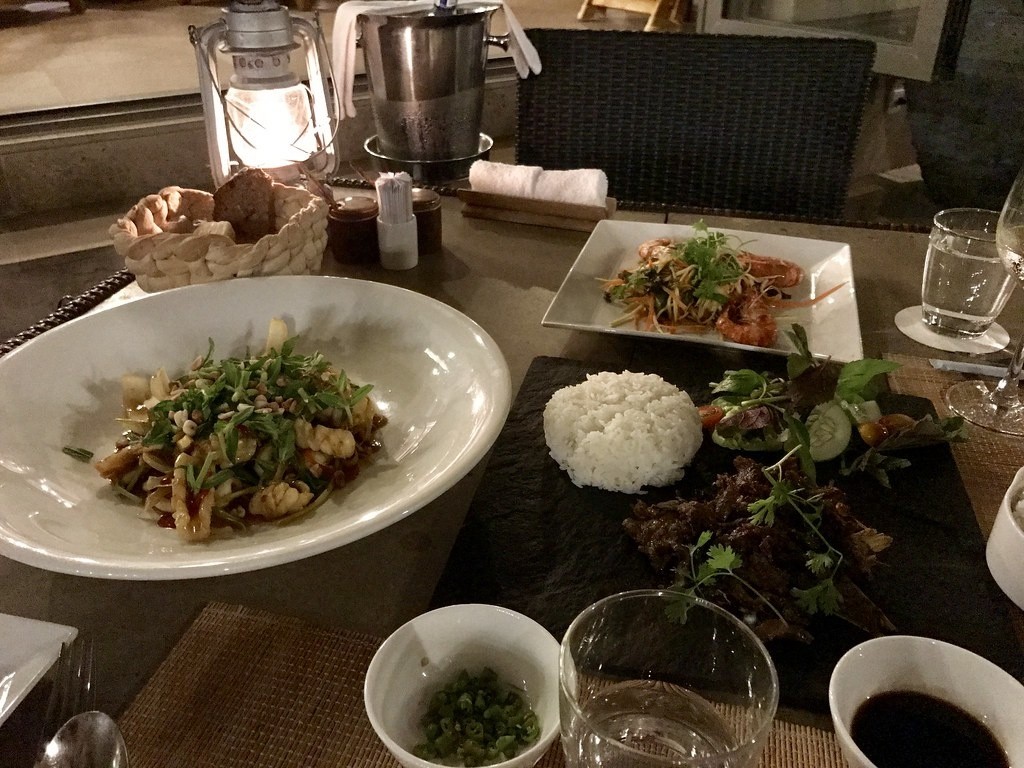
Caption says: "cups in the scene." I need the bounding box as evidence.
[559,589,779,768]
[377,214,418,270]
[921,208,1017,340]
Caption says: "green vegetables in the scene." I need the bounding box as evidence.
[665,321,965,626]
[138,334,375,489]
[612,218,738,304]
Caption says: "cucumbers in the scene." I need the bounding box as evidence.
[785,402,853,477]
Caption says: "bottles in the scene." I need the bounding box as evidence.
[328,196,378,264]
[411,188,442,253]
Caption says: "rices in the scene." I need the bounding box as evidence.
[543,369,703,493]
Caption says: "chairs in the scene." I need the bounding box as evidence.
[514,28,876,222]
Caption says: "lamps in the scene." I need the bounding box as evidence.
[188,0,342,191]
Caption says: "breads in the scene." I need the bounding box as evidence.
[210,166,275,241]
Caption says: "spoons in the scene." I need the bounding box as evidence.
[39,711,129,768]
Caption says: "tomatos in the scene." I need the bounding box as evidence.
[698,406,722,427]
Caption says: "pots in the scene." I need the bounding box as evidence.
[355,0,510,160]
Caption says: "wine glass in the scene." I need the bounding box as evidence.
[946,164,1024,437]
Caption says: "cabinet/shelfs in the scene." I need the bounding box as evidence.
[0,0,689,343]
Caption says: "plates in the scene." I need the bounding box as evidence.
[429,355,1024,733]
[0,613,78,727]
[541,220,865,364]
[0,276,512,581]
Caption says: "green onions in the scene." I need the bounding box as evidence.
[413,666,540,766]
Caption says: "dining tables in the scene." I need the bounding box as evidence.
[0,179,1024,768]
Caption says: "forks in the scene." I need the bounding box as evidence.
[33,639,96,768]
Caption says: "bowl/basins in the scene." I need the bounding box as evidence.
[986,466,1024,612]
[364,604,561,768]
[829,636,1024,768]
